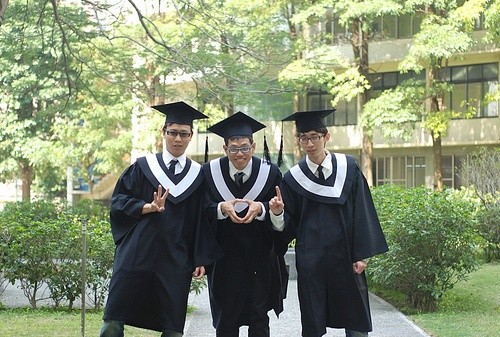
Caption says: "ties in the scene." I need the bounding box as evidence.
[169,160,179,175]
[234,173,246,186]
[316,166,324,180]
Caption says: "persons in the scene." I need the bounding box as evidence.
[101,101,209,337]
[200,111,289,337]
[267,108,389,337]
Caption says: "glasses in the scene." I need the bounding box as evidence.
[226,142,255,154]
[298,134,325,144]
[164,128,192,138]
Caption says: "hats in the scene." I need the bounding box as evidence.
[207,111,266,140]
[281,109,337,132]
[150,101,209,128]
